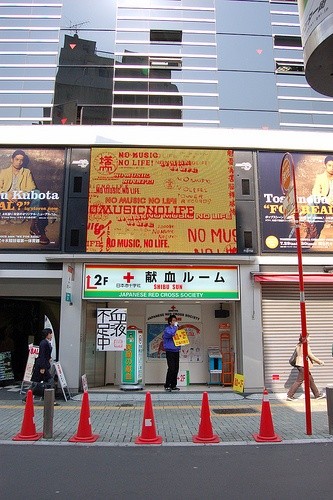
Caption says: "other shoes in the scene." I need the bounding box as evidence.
[171,386,180,390]
[166,387,170,389]
[287,395,298,400]
[22,397,26,402]
[54,400,60,406]
[317,393,326,399]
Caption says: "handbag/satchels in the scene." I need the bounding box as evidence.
[289,348,297,366]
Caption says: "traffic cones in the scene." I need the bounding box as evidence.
[68,391,100,443]
[252,389,283,442]
[11,389,45,441]
[134,391,162,445]
[192,391,221,443]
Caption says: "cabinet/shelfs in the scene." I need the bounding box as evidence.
[221,352,234,388]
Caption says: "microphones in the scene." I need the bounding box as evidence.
[177,324,180,328]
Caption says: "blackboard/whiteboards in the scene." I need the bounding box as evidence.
[0,350,14,381]
[23,346,40,383]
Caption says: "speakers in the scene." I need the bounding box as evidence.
[215,310,229,318]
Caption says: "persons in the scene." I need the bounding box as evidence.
[22,328,60,406]
[287,332,326,401]
[301,156,333,247]
[0,151,50,244]
[163,315,182,392]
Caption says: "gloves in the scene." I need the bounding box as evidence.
[174,322,178,326]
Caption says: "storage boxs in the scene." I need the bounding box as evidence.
[177,370,189,387]
